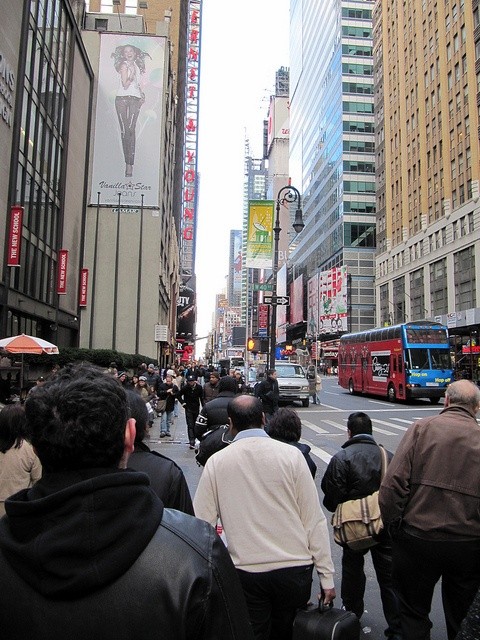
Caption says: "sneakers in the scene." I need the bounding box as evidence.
[160,431,166,438]
[166,433,171,436]
[189,444,195,449]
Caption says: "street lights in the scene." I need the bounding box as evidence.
[266,182,306,371]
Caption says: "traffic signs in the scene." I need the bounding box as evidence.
[263,295,290,306]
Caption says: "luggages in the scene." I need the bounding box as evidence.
[293,597,361,640]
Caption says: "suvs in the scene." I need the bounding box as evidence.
[257,362,317,407]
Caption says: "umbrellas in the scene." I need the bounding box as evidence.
[0,333,60,388]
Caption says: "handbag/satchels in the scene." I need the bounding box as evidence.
[333,490,386,550]
[195,425,232,466]
[157,400,166,413]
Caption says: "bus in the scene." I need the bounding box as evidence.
[338,318,454,404]
[225,355,245,378]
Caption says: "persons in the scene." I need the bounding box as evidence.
[206,372,220,402]
[311,371,321,405]
[320,412,402,640]
[220,366,227,376]
[150,385,159,400]
[257,370,279,414]
[189,362,199,375]
[166,364,170,368]
[119,372,125,382]
[164,370,180,417]
[180,365,185,375]
[128,376,138,386]
[136,363,147,376]
[110,43,152,178]
[0,361,256,639]
[123,389,195,517]
[176,375,205,449]
[142,364,162,390]
[234,371,243,393]
[254,373,264,394]
[378,379,480,640]
[229,369,235,377]
[266,408,317,479]
[324,366,339,376]
[170,364,175,369]
[195,395,337,640]
[135,376,153,437]
[185,364,192,374]
[157,376,179,437]
[195,377,237,439]
[107,364,118,376]
[1,405,44,515]
[197,365,205,390]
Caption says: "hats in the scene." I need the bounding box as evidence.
[117,371,124,377]
[235,371,241,376]
[148,363,154,368]
[210,372,220,380]
[187,375,196,381]
[167,370,176,377]
[139,376,148,381]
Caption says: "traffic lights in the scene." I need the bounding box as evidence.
[246,336,254,352]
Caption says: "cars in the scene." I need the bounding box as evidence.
[243,366,262,389]
